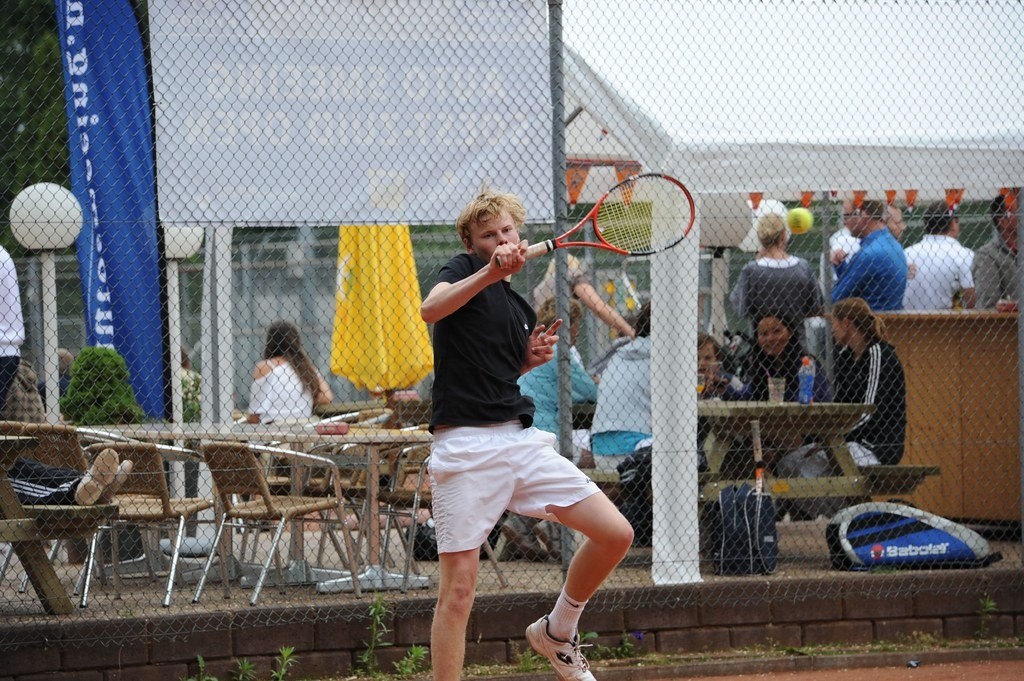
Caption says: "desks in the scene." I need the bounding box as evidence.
[73,421,433,595]
[0,435,74,616]
[867,311,1024,537]
[698,399,875,477]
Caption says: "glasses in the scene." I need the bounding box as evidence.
[843,213,860,220]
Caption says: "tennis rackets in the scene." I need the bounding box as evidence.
[495,172,695,269]
[750,420,766,493]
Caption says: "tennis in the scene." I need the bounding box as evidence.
[787,208,814,235]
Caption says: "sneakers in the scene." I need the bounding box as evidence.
[525,615,597,681]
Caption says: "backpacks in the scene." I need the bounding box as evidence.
[719,482,778,575]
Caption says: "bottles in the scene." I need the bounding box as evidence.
[952,273,963,310]
[798,356,815,405]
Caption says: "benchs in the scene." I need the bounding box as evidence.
[578,462,939,562]
[18,497,119,540]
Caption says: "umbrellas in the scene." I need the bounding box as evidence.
[329,225,432,479]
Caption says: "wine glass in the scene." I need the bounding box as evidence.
[697,374,706,401]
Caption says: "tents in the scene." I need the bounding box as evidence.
[149,1,1024,587]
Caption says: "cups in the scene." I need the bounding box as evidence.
[963,288,976,309]
[768,378,786,406]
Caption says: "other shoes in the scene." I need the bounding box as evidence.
[98,459,133,504]
[74,449,119,506]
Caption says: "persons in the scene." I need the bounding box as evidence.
[9,448,134,505]
[729,215,824,352]
[0,245,26,421]
[815,200,915,310]
[421,194,633,681]
[699,308,831,476]
[901,200,977,308]
[778,297,907,520]
[36,347,73,408]
[505,295,598,562]
[591,302,651,491]
[247,323,333,423]
[976,196,1016,307]
[532,254,634,340]
[181,350,202,404]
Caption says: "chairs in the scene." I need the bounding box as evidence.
[0,408,511,609]
